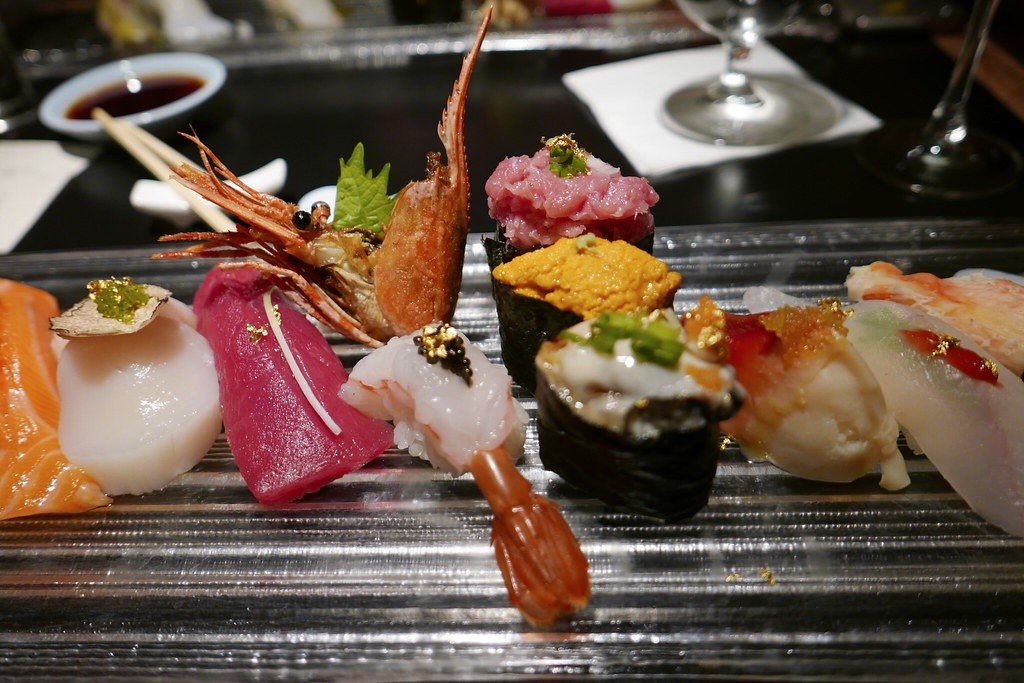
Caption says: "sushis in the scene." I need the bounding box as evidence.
[479,133,747,524]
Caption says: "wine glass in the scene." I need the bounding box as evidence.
[662,0,837,156]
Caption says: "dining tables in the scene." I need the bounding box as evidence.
[0,9,1024,681]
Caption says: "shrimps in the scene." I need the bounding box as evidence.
[152,5,593,633]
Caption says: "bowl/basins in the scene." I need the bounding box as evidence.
[37,53,223,139]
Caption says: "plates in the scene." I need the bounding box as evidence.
[0,211,1022,678]
